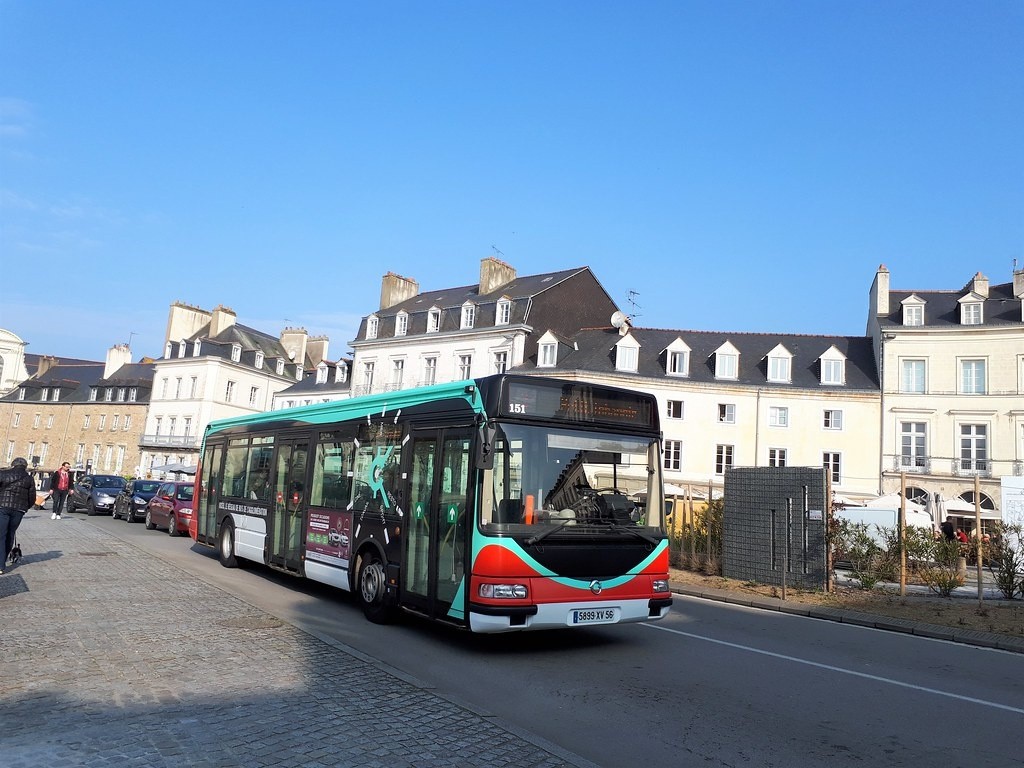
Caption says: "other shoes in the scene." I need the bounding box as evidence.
[0,569,5,575]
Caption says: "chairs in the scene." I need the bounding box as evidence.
[234,471,373,510]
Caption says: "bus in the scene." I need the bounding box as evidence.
[187,373,676,637]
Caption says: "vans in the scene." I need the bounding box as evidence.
[641,499,723,540]
[832,507,937,572]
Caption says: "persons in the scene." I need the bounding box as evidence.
[955,528,968,543]
[0,458,36,574]
[981,531,1002,544]
[249,458,269,501]
[49,461,74,519]
[941,516,956,540]
[934,530,943,542]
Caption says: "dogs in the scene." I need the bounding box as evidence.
[8,543,23,565]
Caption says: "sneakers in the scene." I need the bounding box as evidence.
[57,515,61,519]
[51,513,57,519]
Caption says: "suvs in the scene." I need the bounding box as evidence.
[339,532,349,546]
[327,528,341,546]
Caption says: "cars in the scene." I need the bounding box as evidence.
[145,482,195,537]
[66,474,128,516]
[114,479,165,523]
